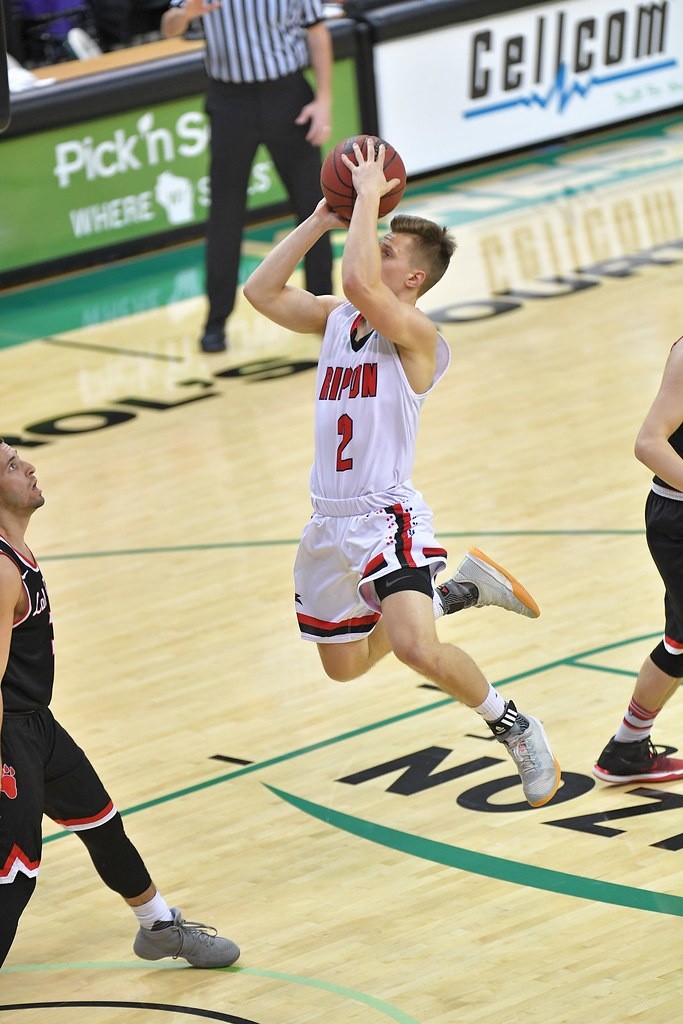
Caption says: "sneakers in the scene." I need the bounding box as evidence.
[133,908,240,968]
[483,700,561,807]
[453,546,541,618]
[592,735,683,783]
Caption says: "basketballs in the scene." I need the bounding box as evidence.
[319,133,407,221]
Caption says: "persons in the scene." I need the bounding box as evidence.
[243,138,561,805]
[0,432,240,970]
[17,0,101,64]
[592,336,683,783]
[161,0,332,352]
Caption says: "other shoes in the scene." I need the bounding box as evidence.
[202,326,225,352]
